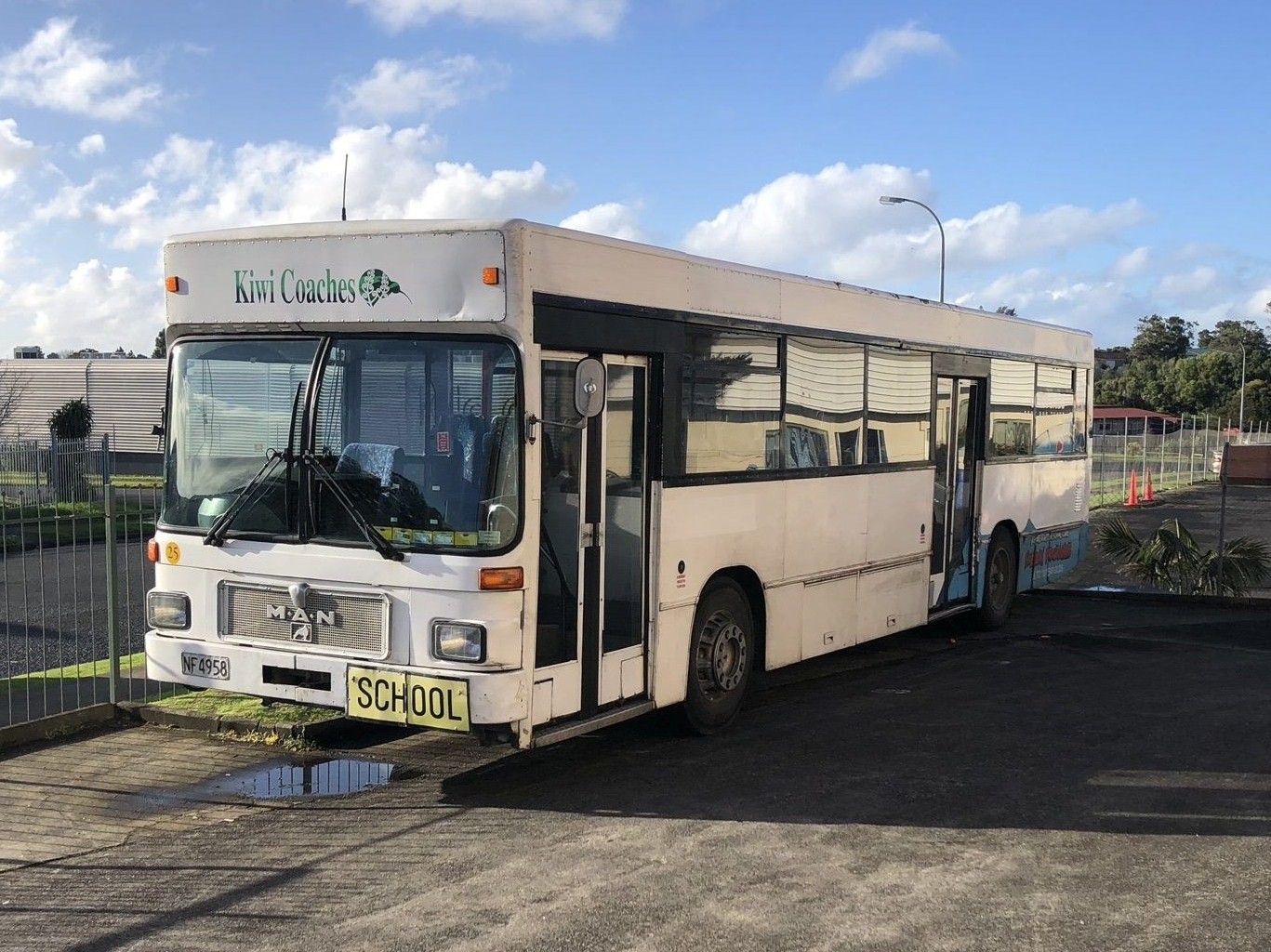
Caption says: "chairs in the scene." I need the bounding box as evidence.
[426,415,485,498]
[334,442,405,497]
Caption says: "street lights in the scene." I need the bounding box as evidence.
[880,196,944,302]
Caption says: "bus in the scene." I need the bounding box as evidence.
[145,218,1093,752]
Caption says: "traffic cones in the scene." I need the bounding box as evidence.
[1140,467,1156,501]
[1123,470,1140,507]
